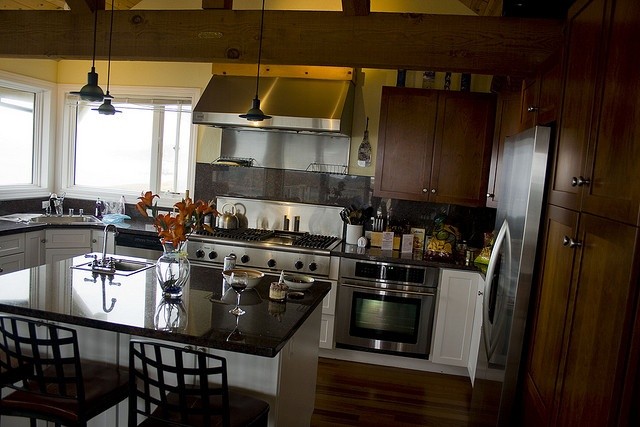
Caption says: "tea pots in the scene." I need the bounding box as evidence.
[212,203,240,229]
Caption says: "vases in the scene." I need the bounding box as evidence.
[153,300,189,336]
[156,239,190,299]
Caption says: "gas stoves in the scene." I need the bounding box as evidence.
[186,228,341,280]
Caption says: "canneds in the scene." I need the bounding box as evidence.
[222,278,231,295]
[463,249,471,265]
[471,252,473,263]
[223,254,236,271]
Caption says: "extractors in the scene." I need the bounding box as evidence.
[192,77,351,138]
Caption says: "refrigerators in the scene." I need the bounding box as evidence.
[468,125,551,416]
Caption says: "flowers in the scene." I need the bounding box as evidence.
[135,190,221,245]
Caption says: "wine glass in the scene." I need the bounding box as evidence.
[227,315,248,345]
[229,271,249,315]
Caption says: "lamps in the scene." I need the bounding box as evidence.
[69,1,115,106]
[90,0,122,116]
[238,1,273,126]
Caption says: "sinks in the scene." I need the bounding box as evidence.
[87,259,147,271]
[32,215,95,224]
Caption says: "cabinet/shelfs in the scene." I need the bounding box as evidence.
[373,86,497,209]
[1,228,25,257]
[431,269,485,375]
[519,66,560,130]
[26,226,46,267]
[527,204,635,427]
[119,330,279,427]
[46,224,91,263]
[0,254,24,276]
[0,313,119,426]
[501,68,524,136]
[547,0,640,227]
[92,225,115,256]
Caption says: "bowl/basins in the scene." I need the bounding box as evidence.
[287,289,313,301]
[280,274,316,290]
[221,269,265,290]
[221,286,263,305]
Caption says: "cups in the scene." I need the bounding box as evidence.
[346,224,363,245]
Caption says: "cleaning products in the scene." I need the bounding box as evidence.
[119,195,125,222]
[94,197,102,218]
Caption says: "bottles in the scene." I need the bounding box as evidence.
[267,299,286,317]
[269,282,288,302]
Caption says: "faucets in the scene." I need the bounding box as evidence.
[101,222,118,265]
[49,194,60,216]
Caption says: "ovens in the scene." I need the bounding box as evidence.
[334,252,440,361]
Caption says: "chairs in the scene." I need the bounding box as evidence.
[0,347,41,426]
[0,317,135,427]
[128,339,270,427]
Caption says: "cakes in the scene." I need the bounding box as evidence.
[268,269,288,301]
